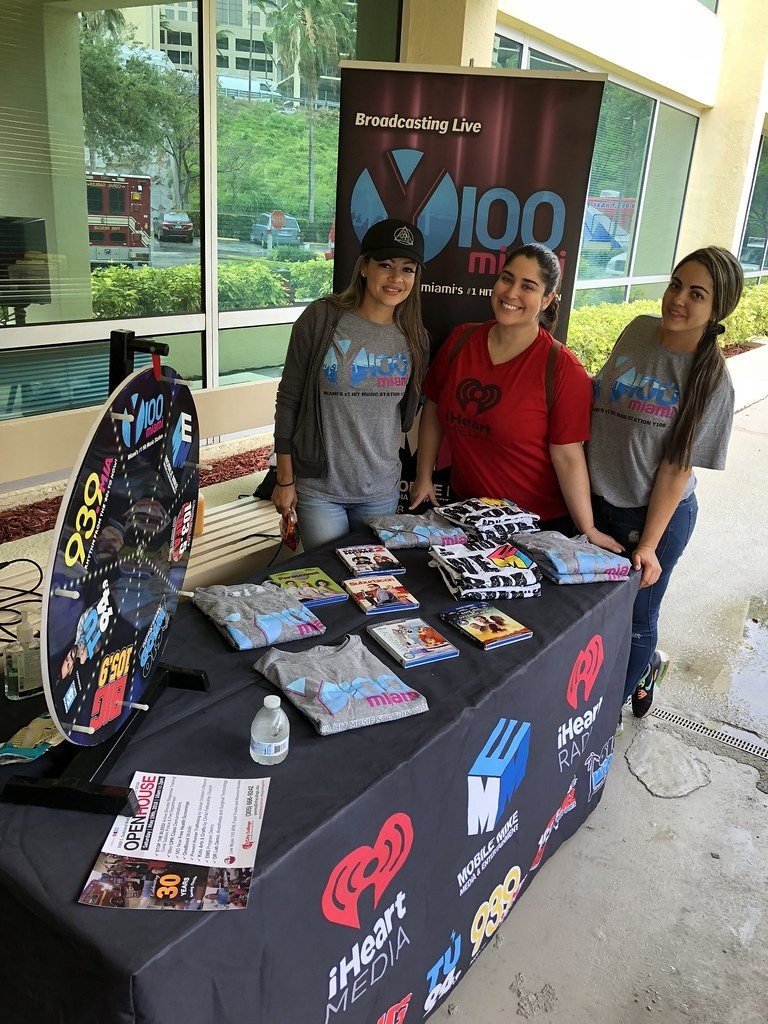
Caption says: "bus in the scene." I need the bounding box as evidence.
[115,44,177,85]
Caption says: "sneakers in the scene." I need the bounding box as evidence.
[614,711,623,736]
[631,650,669,718]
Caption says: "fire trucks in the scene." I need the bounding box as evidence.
[86,171,154,269]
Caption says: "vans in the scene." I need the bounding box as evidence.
[742,240,768,272]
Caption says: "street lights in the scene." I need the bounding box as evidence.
[608,114,637,251]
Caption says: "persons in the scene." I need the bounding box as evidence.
[150,864,252,909]
[271,219,429,553]
[469,614,509,634]
[573,246,744,737]
[58,598,109,681]
[284,580,342,603]
[350,550,402,571]
[360,583,413,607]
[408,243,626,553]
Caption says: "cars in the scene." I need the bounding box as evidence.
[604,251,629,278]
[152,209,195,244]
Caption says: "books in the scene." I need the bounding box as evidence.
[268,567,349,608]
[340,575,420,615]
[366,616,460,668]
[440,601,534,652]
[335,545,406,577]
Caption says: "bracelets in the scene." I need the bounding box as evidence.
[275,476,296,486]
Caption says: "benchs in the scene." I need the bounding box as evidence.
[0,495,304,660]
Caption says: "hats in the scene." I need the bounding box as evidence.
[360,219,426,268]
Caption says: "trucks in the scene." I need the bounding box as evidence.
[217,74,283,105]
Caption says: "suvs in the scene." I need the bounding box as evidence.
[249,210,303,249]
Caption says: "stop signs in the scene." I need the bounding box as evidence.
[271,209,286,230]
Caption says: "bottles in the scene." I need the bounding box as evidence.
[193,489,205,536]
[249,695,289,766]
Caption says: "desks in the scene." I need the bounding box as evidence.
[0,503,644,1024]
